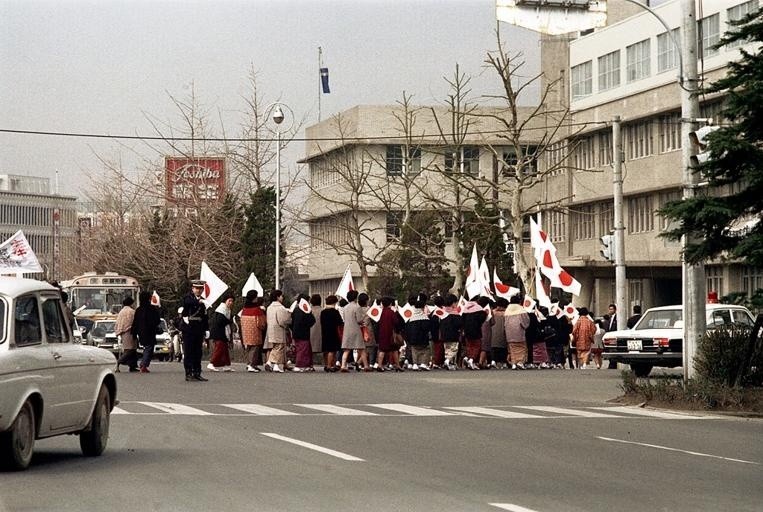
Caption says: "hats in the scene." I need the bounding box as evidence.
[190,280,206,288]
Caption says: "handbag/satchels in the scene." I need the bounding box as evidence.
[156,327,163,334]
[361,327,373,342]
[391,333,404,346]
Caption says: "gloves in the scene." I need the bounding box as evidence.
[205,331,210,339]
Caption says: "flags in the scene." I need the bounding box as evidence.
[334,269,354,304]
[199,261,228,310]
[465,217,581,308]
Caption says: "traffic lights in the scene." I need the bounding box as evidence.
[689,126,730,167]
[600,235,615,264]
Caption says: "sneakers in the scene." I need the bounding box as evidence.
[128,362,235,382]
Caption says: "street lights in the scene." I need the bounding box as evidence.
[264,104,296,294]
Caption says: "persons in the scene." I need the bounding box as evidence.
[205,290,617,372]
[117,282,208,382]
[627,305,643,329]
[84,295,96,310]
[28,278,74,342]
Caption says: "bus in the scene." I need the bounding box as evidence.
[59,272,146,342]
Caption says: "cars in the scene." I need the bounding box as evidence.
[86,320,122,359]
[0,278,120,469]
[600,304,762,376]
[138,317,171,361]
